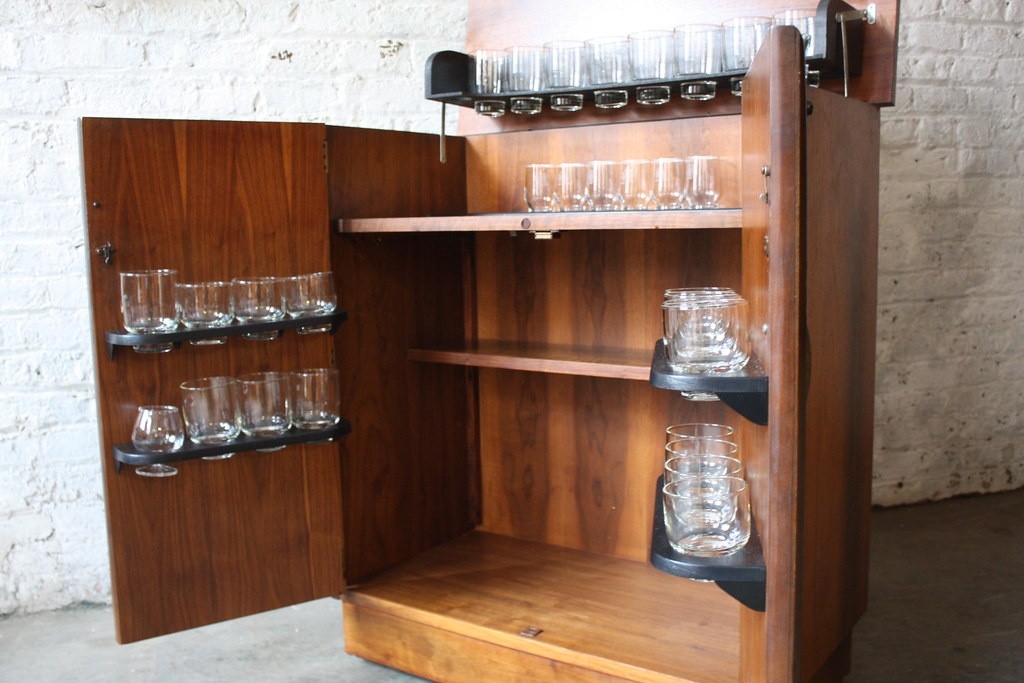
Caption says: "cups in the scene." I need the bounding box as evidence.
[179,368,339,460]
[659,285,753,401]
[524,156,723,211]
[120,268,337,353]
[467,8,816,117]
[659,422,751,583]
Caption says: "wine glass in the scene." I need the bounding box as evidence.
[131,405,185,478]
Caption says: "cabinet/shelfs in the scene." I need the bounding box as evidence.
[80,27,881,683]
